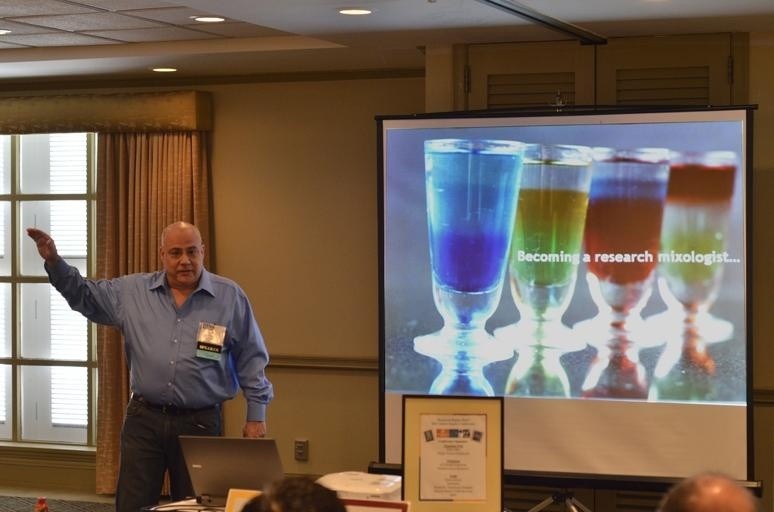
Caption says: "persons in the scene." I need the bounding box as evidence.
[654,470,762,511]
[24,220,274,511]
[240,472,346,510]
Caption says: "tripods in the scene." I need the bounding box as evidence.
[527,490,593,512]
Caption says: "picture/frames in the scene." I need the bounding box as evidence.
[402,394,506,510]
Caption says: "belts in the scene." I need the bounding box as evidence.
[131,393,221,416]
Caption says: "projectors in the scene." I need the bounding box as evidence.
[316,470,402,512]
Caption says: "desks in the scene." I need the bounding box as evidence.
[138,487,557,512]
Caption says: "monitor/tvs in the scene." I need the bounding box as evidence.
[179,436,285,499]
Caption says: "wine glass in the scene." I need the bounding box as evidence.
[413,142,528,363]
[571,151,672,348]
[647,151,738,345]
[494,144,595,353]
